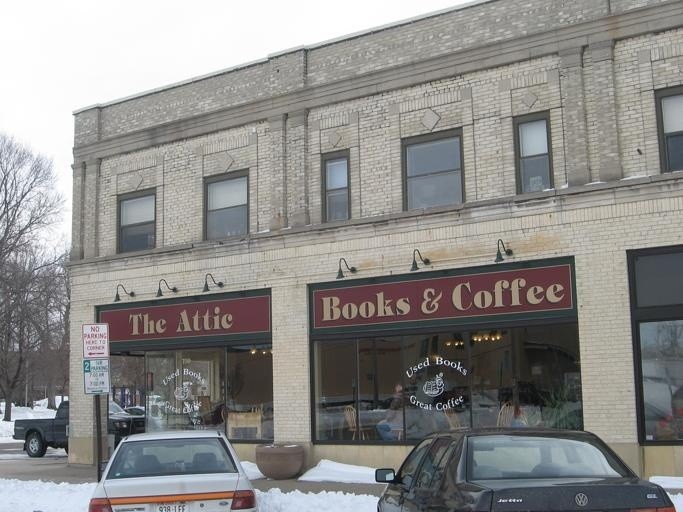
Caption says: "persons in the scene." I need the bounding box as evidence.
[376,385,404,440]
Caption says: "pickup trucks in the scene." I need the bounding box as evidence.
[12,398,144,461]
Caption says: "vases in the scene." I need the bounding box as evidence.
[256,444,304,480]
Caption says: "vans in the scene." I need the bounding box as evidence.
[147,403,167,430]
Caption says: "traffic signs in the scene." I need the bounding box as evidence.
[81,322,109,359]
[82,359,110,396]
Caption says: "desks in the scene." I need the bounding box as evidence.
[226,413,263,440]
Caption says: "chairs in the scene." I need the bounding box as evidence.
[445,408,469,432]
[496,401,512,426]
[135,455,162,472]
[344,405,371,441]
[191,453,217,470]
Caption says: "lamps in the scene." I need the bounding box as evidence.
[495,239,512,262]
[156,279,177,297]
[114,283,134,302]
[336,258,356,279]
[411,249,430,271]
[203,273,223,292]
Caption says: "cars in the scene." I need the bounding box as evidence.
[368,423,679,512]
[122,404,144,415]
[83,428,260,512]
[545,401,681,446]
